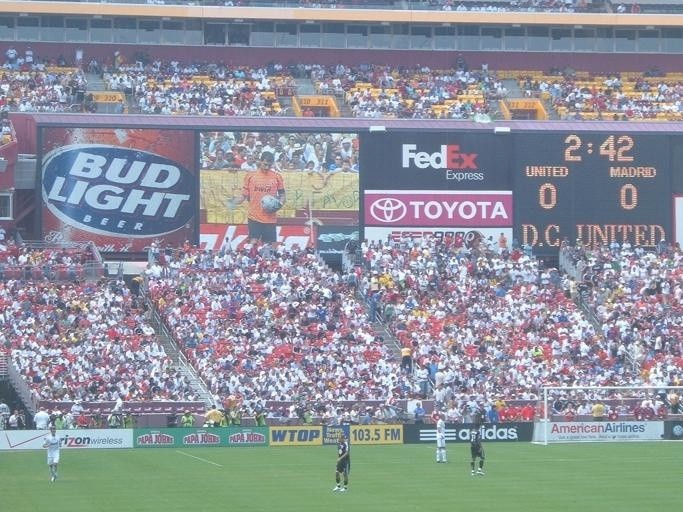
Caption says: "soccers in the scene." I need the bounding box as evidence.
[261,196,278,212]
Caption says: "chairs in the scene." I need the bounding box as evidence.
[582,112,616,121]
[497,70,565,83]
[658,112,681,120]
[431,99,457,117]
[620,72,681,99]
[457,85,484,106]
[575,72,608,92]
[345,81,400,100]
[406,100,415,107]
[46,67,77,74]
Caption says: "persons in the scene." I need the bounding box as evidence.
[470,426,486,475]
[43,426,61,481]
[331,435,351,491]
[435,414,447,463]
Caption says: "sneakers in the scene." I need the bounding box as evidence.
[333,486,341,491]
[476,470,485,475]
[50,472,58,482]
[472,471,476,475]
[339,487,348,492]
